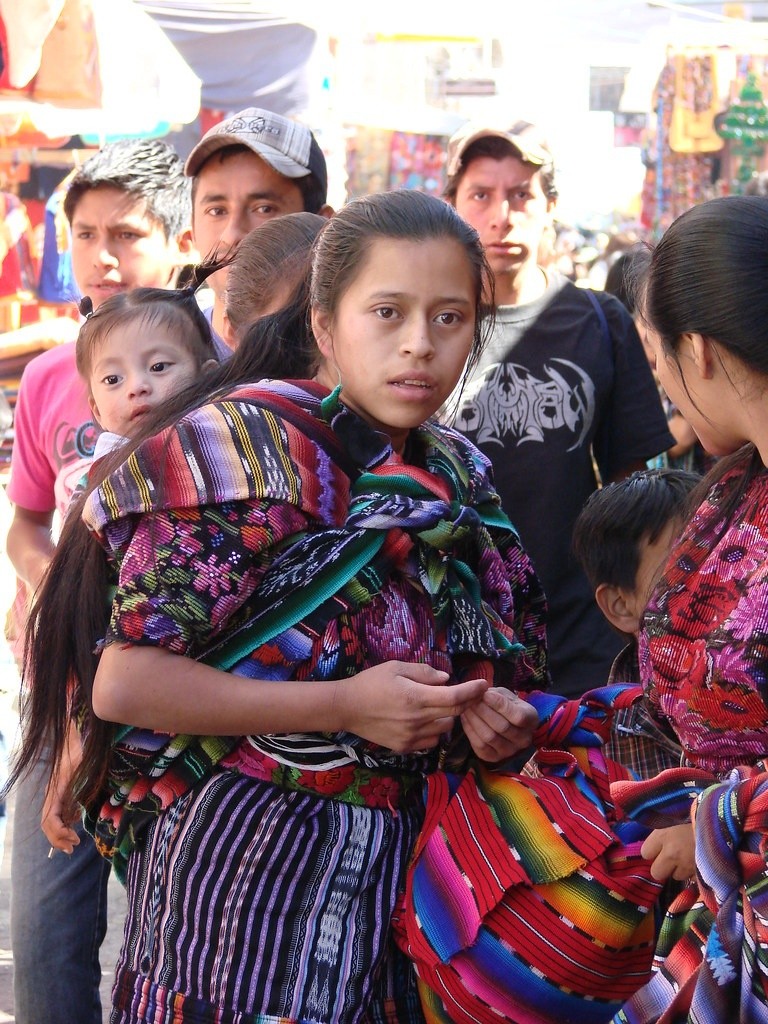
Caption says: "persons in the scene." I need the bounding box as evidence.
[0,193,549,1024]
[572,470,704,783]
[76,261,222,463]
[444,117,679,696]
[540,224,724,474]
[636,194,768,1024]
[4,108,333,1024]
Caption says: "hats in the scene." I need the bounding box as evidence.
[446,115,556,176]
[184,106,328,202]
